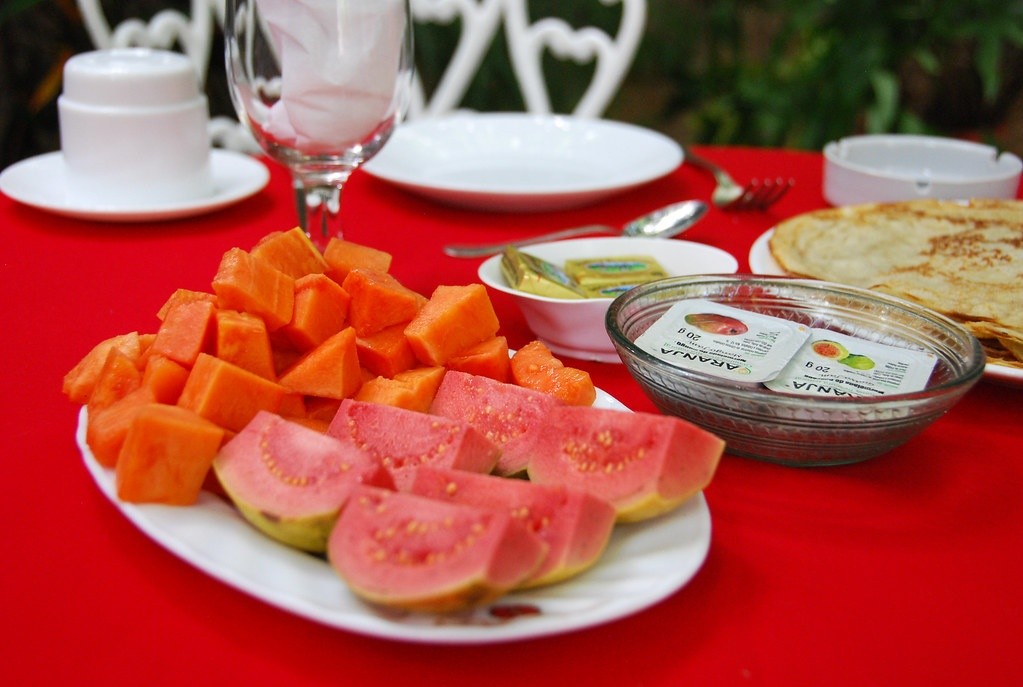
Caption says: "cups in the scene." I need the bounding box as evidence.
[58,47,212,204]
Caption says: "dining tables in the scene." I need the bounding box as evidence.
[2,142,1023,687]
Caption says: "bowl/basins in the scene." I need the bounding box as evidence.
[604,273,988,467]
[475,234,738,362]
[819,131,1023,210]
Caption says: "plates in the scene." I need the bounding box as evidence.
[356,110,685,215]
[73,349,712,646]
[743,224,1023,393]
[0,146,273,226]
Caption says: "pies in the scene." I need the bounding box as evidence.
[770,198,1023,371]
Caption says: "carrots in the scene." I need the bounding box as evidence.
[60,227,596,508]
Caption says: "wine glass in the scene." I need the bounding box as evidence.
[225,0,415,258]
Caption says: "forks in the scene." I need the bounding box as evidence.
[682,145,793,212]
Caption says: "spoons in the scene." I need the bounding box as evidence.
[443,198,707,259]
